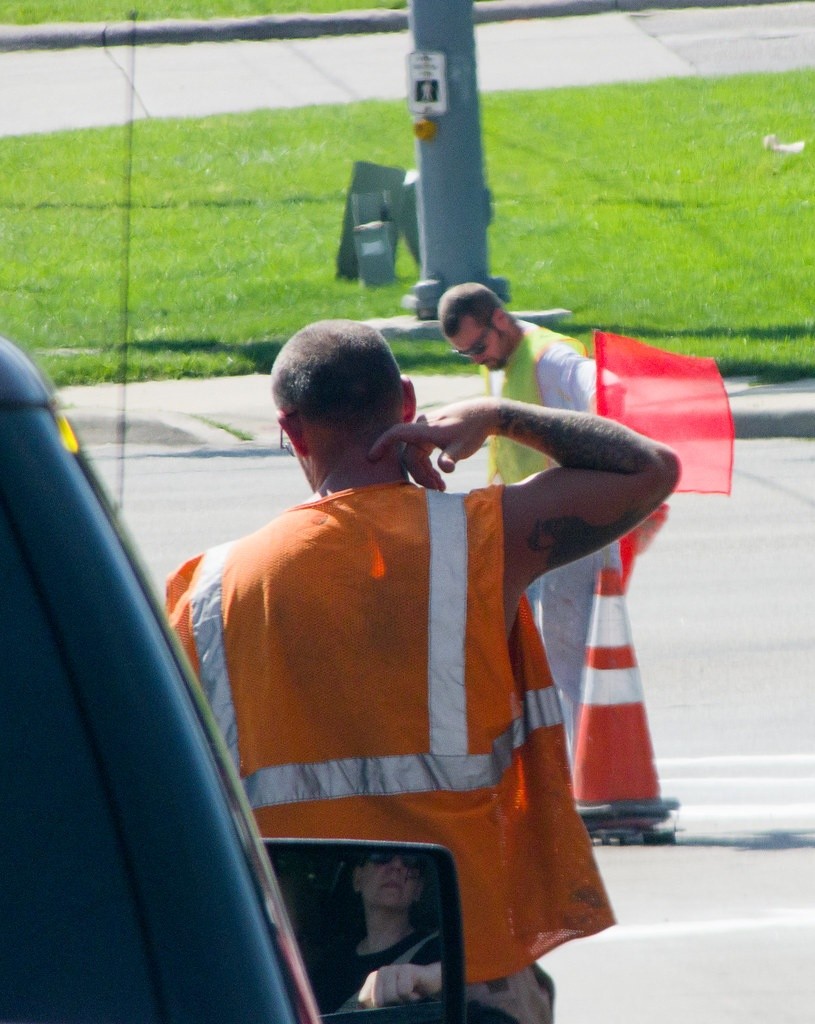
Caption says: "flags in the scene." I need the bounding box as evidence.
[592,328,736,496]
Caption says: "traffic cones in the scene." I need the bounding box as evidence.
[569,567,684,842]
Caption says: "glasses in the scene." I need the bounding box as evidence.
[453,320,490,356]
[280,411,299,457]
[361,849,420,871]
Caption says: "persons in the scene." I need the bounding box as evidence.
[167,321,684,1024]
[437,281,629,772]
[309,848,443,1017]
